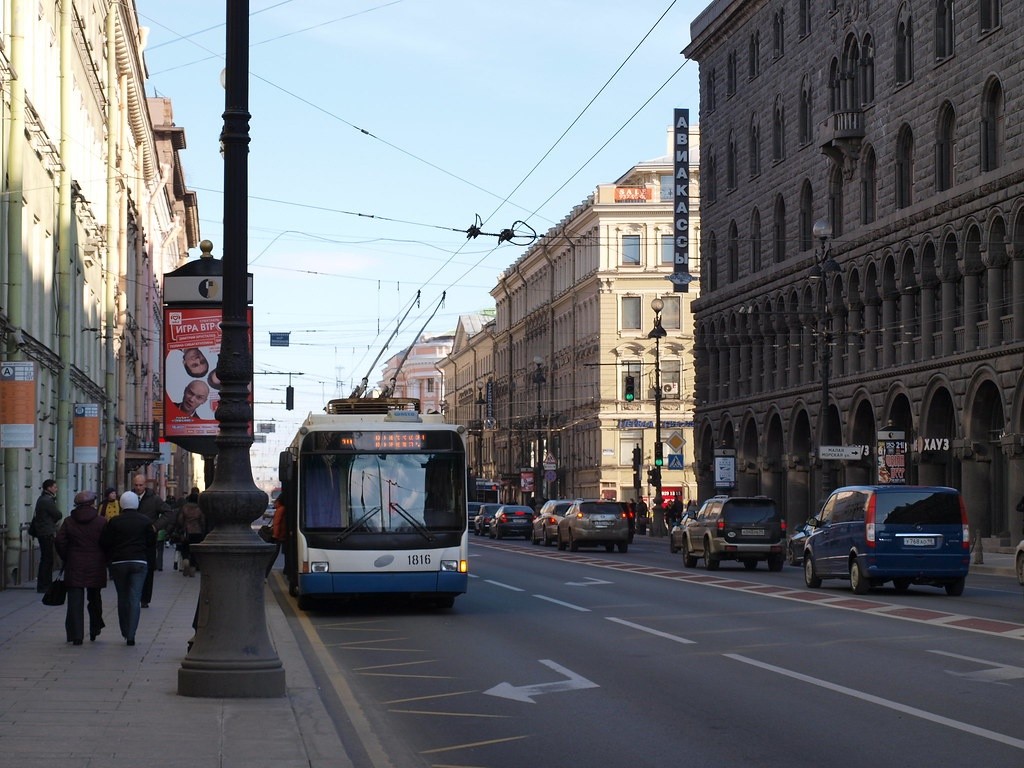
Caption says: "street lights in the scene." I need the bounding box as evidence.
[647,294,668,538]
[474,381,487,477]
[531,353,546,496]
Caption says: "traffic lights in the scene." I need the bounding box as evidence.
[648,469,657,486]
[655,441,664,467]
[632,448,640,471]
[625,375,634,401]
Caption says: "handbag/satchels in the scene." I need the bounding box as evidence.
[258,516,275,541]
[27,516,39,538]
[170,526,187,544]
[41,560,68,606]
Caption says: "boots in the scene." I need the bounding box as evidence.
[189,567,195,578]
[183,559,189,576]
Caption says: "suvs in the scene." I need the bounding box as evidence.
[803,484,972,596]
[530,496,636,553]
[682,494,789,572]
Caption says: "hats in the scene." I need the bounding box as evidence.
[120,491,139,509]
[74,492,97,505]
[106,488,117,497]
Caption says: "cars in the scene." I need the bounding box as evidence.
[787,510,821,565]
[468,502,534,541]
[670,510,698,554]
[262,503,276,521]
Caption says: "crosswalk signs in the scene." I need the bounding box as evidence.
[668,454,684,471]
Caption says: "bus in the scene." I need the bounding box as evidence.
[270,487,282,500]
[277,290,468,612]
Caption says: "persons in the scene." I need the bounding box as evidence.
[33,479,63,593]
[172,380,209,419]
[207,369,221,390]
[56,491,108,645]
[179,347,209,378]
[630,495,647,535]
[121,474,171,607]
[264,498,284,581]
[100,487,203,577]
[664,495,683,535]
[102,491,155,646]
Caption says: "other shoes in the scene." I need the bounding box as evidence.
[90,630,101,641]
[127,637,135,646]
[72,640,82,646]
[174,561,178,571]
[141,603,149,608]
